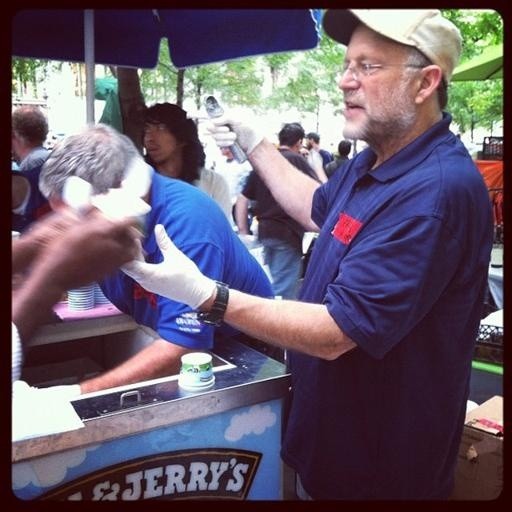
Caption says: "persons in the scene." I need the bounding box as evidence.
[305,133,332,165]
[217,142,253,224]
[236,122,329,303]
[12,196,145,386]
[325,140,352,178]
[142,102,232,231]
[111,7,494,504]
[13,104,54,233]
[35,122,284,402]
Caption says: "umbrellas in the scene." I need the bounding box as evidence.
[12,5,322,126]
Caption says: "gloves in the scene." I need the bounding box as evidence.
[119,224,217,311]
[187,110,266,155]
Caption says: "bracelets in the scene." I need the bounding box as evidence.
[199,278,229,327]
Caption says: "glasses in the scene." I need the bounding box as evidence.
[330,63,425,84]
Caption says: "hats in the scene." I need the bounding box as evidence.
[322,9,463,87]
